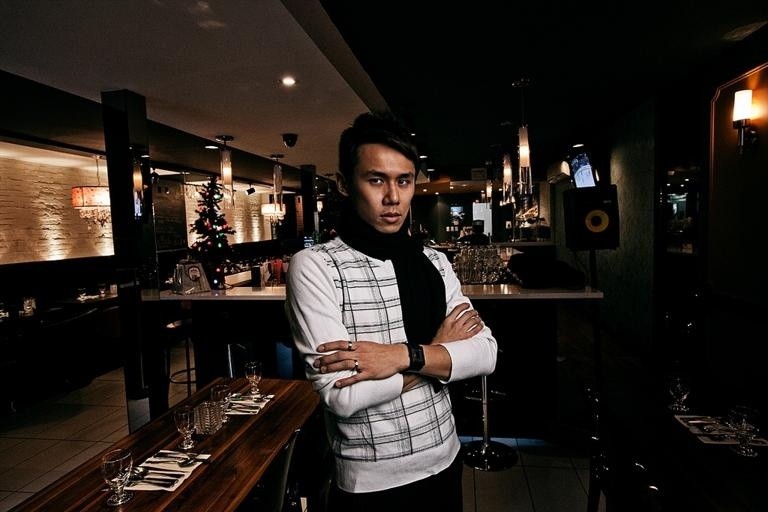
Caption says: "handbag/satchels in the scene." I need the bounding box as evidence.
[171,259,212,295]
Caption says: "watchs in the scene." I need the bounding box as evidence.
[397,341,426,374]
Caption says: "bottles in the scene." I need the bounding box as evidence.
[456,245,507,285]
[173,404,199,450]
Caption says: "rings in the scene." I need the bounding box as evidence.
[351,359,358,372]
[347,341,353,351]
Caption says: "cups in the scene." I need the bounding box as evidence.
[23,296,32,314]
[193,401,223,435]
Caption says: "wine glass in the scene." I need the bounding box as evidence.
[101,446,134,506]
[666,377,692,412]
[211,385,231,424]
[726,404,760,458]
[244,360,264,394]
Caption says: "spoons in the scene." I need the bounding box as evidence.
[130,452,201,489]
[223,392,268,414]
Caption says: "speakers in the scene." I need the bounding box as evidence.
[564,184,619,251]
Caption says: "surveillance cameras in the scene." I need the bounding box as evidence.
[283,135,296,148]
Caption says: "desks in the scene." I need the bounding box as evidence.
[55,292,118,308]
[674,413,768,461]
[2,377,322,512]
[0,300,41,326]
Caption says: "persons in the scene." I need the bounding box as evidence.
[283,111,498,510]
[451,219,489,246]
[505,224,513,243]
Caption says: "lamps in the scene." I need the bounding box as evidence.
[732,89,759,158]
[261,154,287,227]
[215,135,236,210]
[157,182,170,195]
[133,144,150,159]
[131,142,146,218]
[71,154,112,210]
[245,182,255,196]
[479,77,534,208]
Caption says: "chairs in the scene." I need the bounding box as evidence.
[582,383,715,512]
[238,429,308,512]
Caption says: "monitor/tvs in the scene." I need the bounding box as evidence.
[563,139,600,187]
